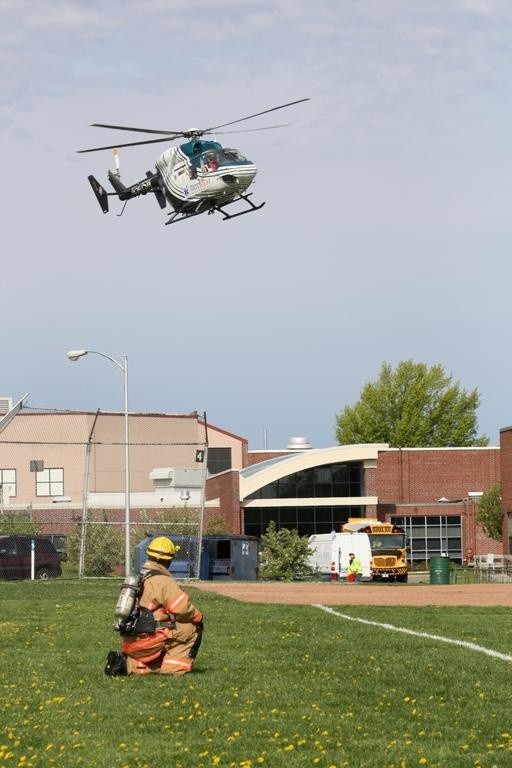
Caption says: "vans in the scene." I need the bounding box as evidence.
[467,551,512,574]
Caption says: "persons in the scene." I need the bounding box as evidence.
[348,552,363,583]
[105,535,205,679]
[207,153,218,168]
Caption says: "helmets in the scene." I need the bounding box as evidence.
[146,536,175,560]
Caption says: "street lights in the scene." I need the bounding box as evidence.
[67,347,131,582]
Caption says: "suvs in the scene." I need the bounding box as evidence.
[1,534,65,583]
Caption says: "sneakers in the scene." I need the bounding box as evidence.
[105,651,127,676]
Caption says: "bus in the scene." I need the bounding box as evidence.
[342,516,408,583]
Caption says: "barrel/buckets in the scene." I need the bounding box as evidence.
[429,556,450,584]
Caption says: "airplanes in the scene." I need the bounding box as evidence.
[68,98,316,223]
